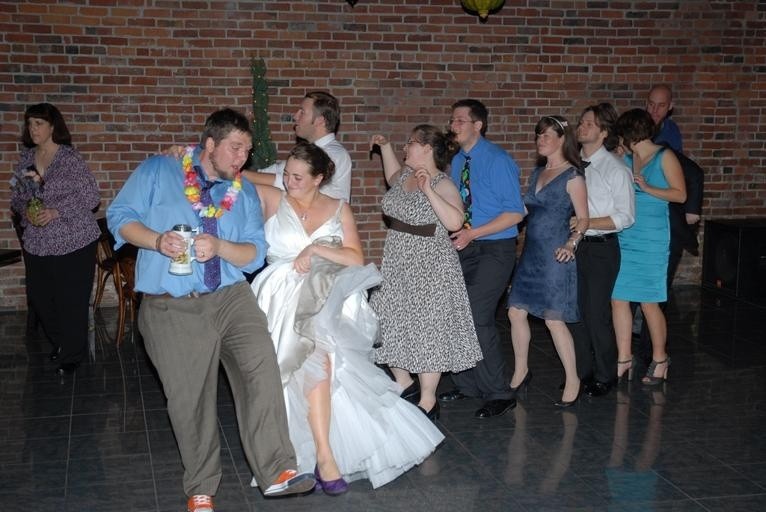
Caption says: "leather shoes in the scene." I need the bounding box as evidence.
[474,397,517,419]
[557,377,590,390]
[586,372,620,399]
[48,345,62,361]
[416,401,440,420]
[440,388,468,402]
[54,358,80,371]
[401,381,420,403]
[314,462,348,495]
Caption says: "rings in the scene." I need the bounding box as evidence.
[563,252,567,255]
[456,244,461,247]
[201,252,205,257]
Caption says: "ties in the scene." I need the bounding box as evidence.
[461,157,474,231]
[200,183,222,291]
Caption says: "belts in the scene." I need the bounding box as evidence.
[141,290,207,300]
[584,233,617,241]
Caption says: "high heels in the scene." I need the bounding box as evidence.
[642,356,672,386]
[507,367,533,396]
[617,356,636,381]
[553,378,585,407]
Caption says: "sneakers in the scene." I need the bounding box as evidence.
[264,468,315,498]
[187,494,213,512]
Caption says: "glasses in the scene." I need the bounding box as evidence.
[447,119,474,124]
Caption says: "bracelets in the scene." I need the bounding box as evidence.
[573,229,586,237]
[570,237,578,251]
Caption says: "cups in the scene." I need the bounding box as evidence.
[169,224,200,275]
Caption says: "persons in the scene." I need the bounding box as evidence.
[612,106,687,386]
[160,142,364,497]
[105,107,316,512]
[439,101,525,420]
[640,155,703,358]
[560,103,636,398]
[9,104,102,379]
[509,115,588,409]
[646,87,686,156]
[366,125,483,423]
[238,92,351,209]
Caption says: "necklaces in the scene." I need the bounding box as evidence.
[544,160,569,170]
[292,202,315,221]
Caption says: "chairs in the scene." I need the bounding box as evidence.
[112,243,142,348]
[91,217,146,323]
